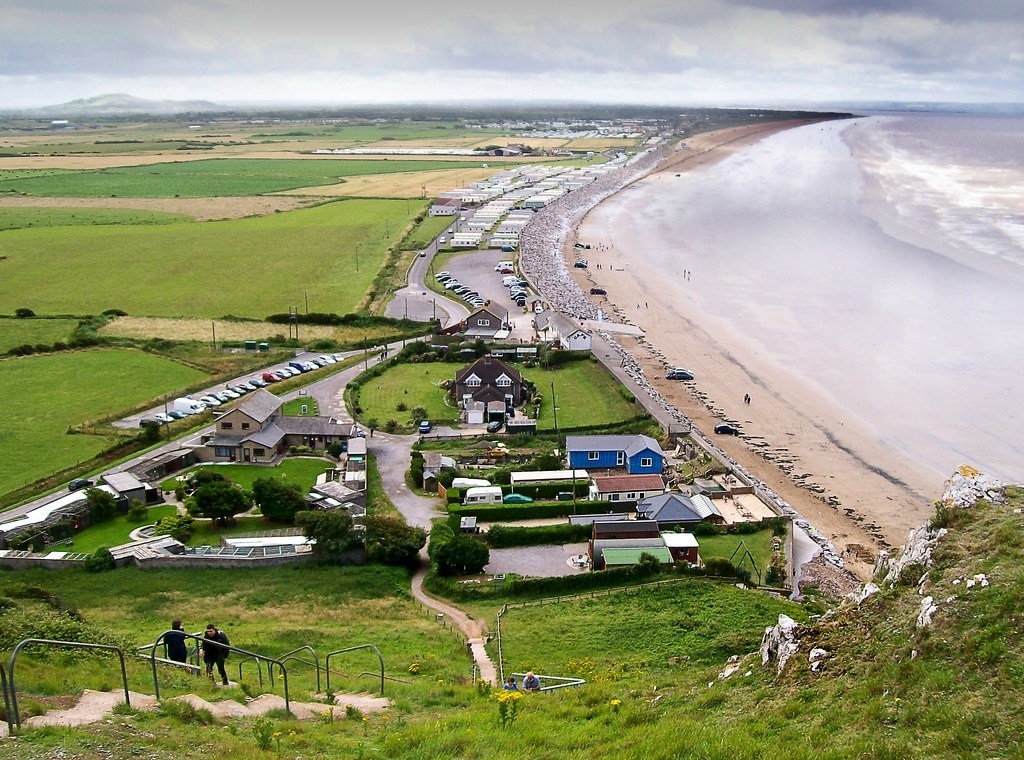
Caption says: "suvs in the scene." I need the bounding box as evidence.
[68,479,93,491]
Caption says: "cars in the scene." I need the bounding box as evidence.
[461,217,465,221]
[574,262,587,268]
[418,421,431,433]
[501,269,514,274]
[503,494,533,504]
[714,425,735,435]
[434,271,485,309]
[448,229,453,233]
[674,368,694,379]
[487,421,502,433]
[575,243,584,247]
[586,244,590,249]
[439,237,445,243]
[420,251,426,257]
[139,353,344,427]
[589,288,607,295]
[501,276,529,306]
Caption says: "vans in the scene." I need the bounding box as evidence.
[464,487,503,504]
[494,262,513,271]
[535,306,543,314]
[502,245,516,252]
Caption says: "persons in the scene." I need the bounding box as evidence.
[744,393,751,404]
[523,672,540,693]
[503,676,519,690]
[199,624,230,686]
[163,619,188,668]
